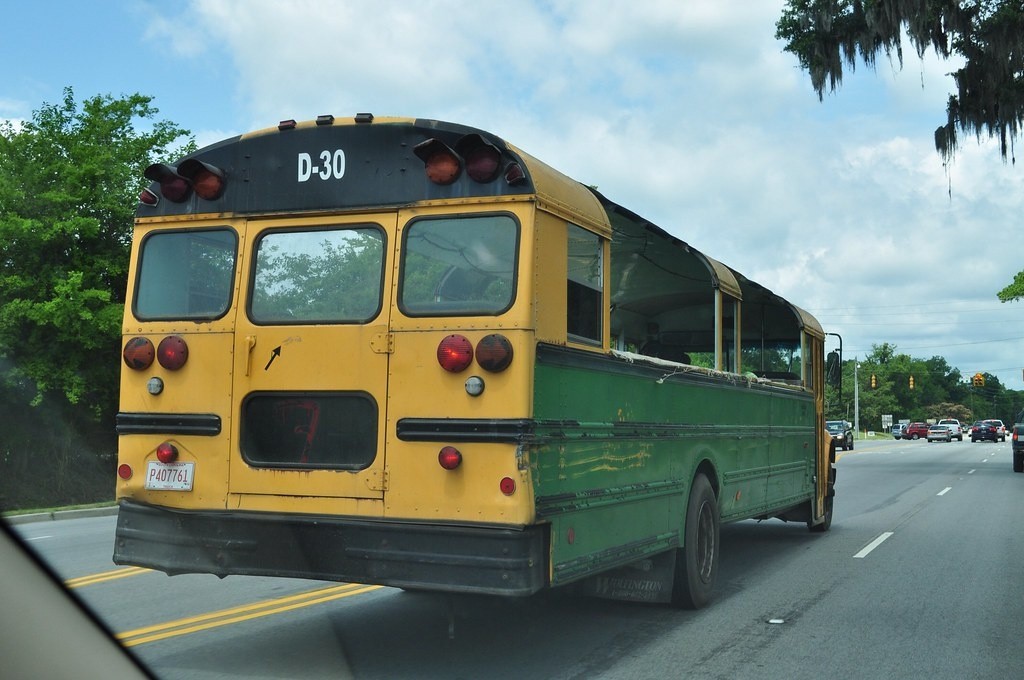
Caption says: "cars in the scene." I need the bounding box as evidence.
[972,421,999,443]
[927,425,952,442]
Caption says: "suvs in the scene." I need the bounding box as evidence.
[825,420,853,450]
[902,423,932,440]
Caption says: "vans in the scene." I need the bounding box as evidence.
[892,424,908,440]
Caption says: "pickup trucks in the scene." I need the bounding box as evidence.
[1012,409,1024,472]
[981,420,1005,442]
[938,419,962,441]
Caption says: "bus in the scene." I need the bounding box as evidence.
[113,113,842,609]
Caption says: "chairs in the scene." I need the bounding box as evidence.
[641,340,692,366]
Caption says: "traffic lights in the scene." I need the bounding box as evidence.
[973,373,984,386]
[870,374,877,389]
[909,375,915,390]
